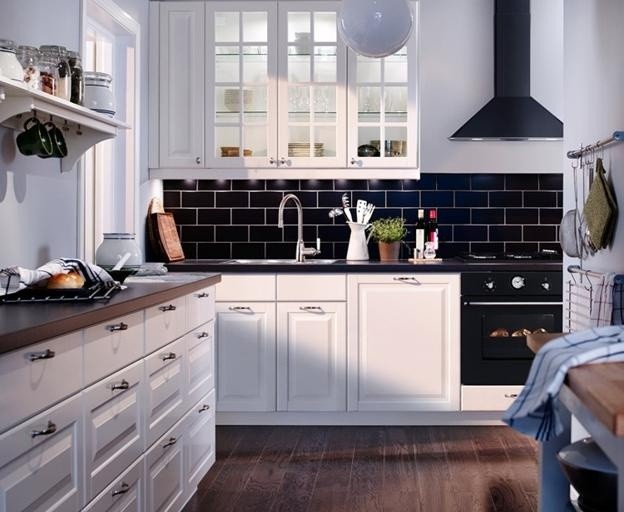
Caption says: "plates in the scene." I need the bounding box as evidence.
[288,141,326,159]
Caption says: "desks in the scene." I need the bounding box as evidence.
[526,329,624,512]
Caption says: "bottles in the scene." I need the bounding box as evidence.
[1,38,117,117]
[414,208,440,259]
[95,234,140,270]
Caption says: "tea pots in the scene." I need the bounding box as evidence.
[345,218,374,262]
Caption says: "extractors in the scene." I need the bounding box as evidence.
[450,1,564,141]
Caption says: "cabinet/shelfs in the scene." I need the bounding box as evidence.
[0,272,222,512]
[149,0,422,180]
[214,274,461,427]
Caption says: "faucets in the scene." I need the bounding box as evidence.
[277,193,322,262]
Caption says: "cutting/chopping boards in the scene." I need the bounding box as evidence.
[152,212,187,262]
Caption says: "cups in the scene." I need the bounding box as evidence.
[18,116,67,161]
[372,140,404,156]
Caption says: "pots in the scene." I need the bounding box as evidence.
[357,144,378,157]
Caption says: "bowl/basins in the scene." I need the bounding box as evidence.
[221,147,252,157]
[224,89,256,113]
[103,266,139,281]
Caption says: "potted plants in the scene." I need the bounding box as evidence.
[369,217,408,261]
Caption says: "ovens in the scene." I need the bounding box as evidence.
[460,299,562,384]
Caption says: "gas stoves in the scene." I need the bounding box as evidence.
[459,251,564,294]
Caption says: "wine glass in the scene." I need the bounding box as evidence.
[289,89,331,117]
[354,89,407,113]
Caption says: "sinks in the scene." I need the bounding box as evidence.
[219,259,345,267]
